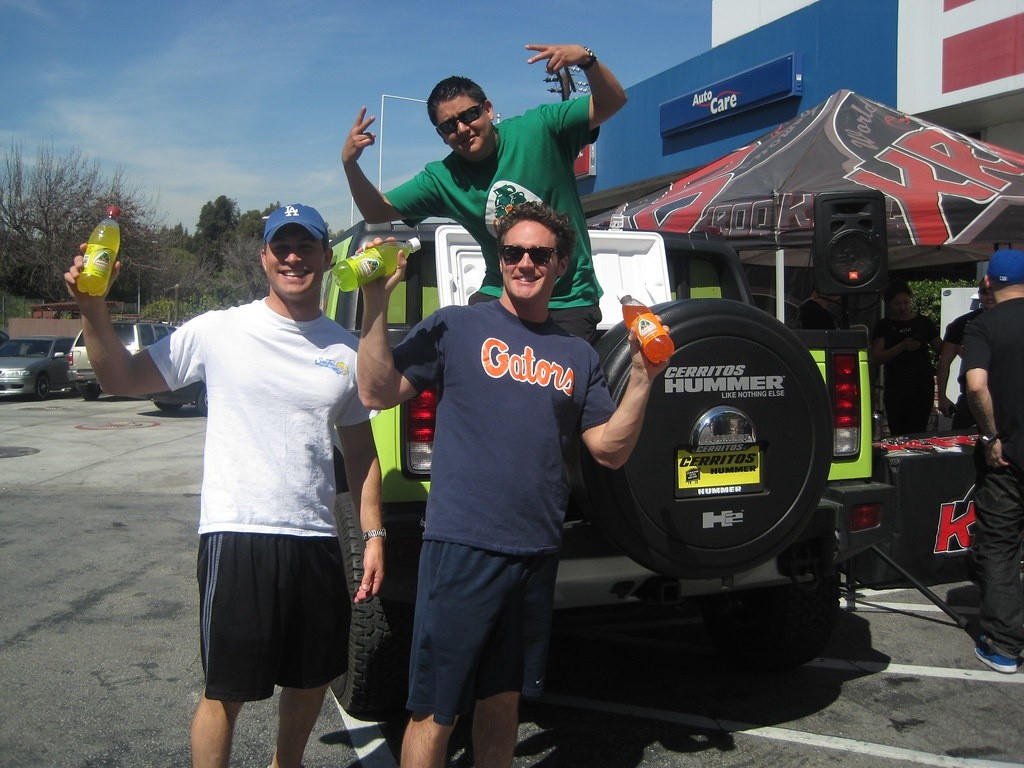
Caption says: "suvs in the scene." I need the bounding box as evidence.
[310,216,889,722]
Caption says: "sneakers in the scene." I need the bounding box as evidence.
[973,643,1018,673]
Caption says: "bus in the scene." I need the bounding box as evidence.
[66,318,178,402]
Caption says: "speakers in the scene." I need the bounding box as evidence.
[814,188,889,296]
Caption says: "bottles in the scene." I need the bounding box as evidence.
[332,237,421,292]
[617,290,675,364]
[77,205,121,296]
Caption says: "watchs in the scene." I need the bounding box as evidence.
[577,45,596,69]
[362,527,387,542]
[979,430,1000,447]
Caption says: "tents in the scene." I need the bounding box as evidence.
[585,88,1024,331]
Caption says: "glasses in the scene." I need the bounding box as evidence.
[978,289,992,296]
[436,101,485,137]
[499,244,559,266]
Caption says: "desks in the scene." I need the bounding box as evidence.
[847,434,976,590]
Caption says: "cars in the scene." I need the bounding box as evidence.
[150,377,208,417]
[0,335,76,401]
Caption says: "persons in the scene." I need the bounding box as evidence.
[64,203,387,768]
[341,44,628,347]
[799,281,851,331]
[937,276,996,430]
[957,248,1024,674]
[356,201,672,768]
[870,279,943,438]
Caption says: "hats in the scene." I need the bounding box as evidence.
[987,248,1024,285]
[264,204,328,250]
